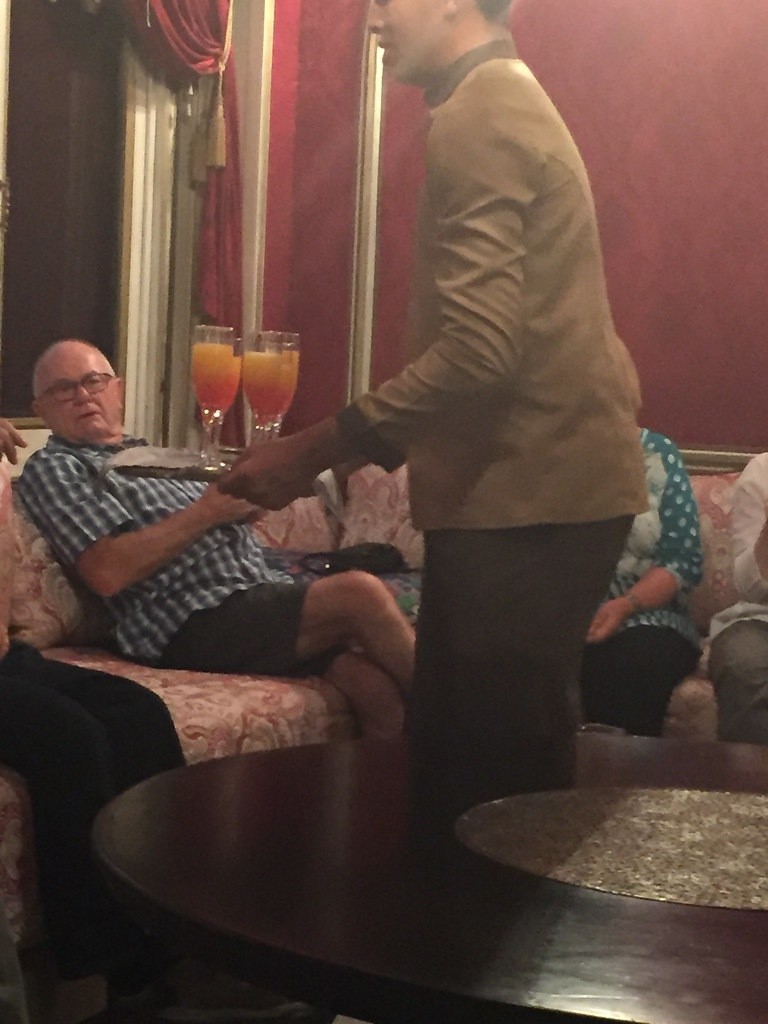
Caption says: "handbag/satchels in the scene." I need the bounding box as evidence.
[294,540,406,576]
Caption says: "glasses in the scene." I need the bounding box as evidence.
[47,371,114,403]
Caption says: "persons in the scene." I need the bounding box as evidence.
[706,450,768,746]
[17,338,410,740]
[214,0,652,737]
[580,426,704,736]
[0,412,190,998]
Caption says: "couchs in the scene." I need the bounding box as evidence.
[0,462,768,961]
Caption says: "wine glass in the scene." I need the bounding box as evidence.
[187,326,301,473]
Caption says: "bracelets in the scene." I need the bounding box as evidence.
[623,593,644,614]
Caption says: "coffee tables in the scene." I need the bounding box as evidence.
[90,732,768,1022]
